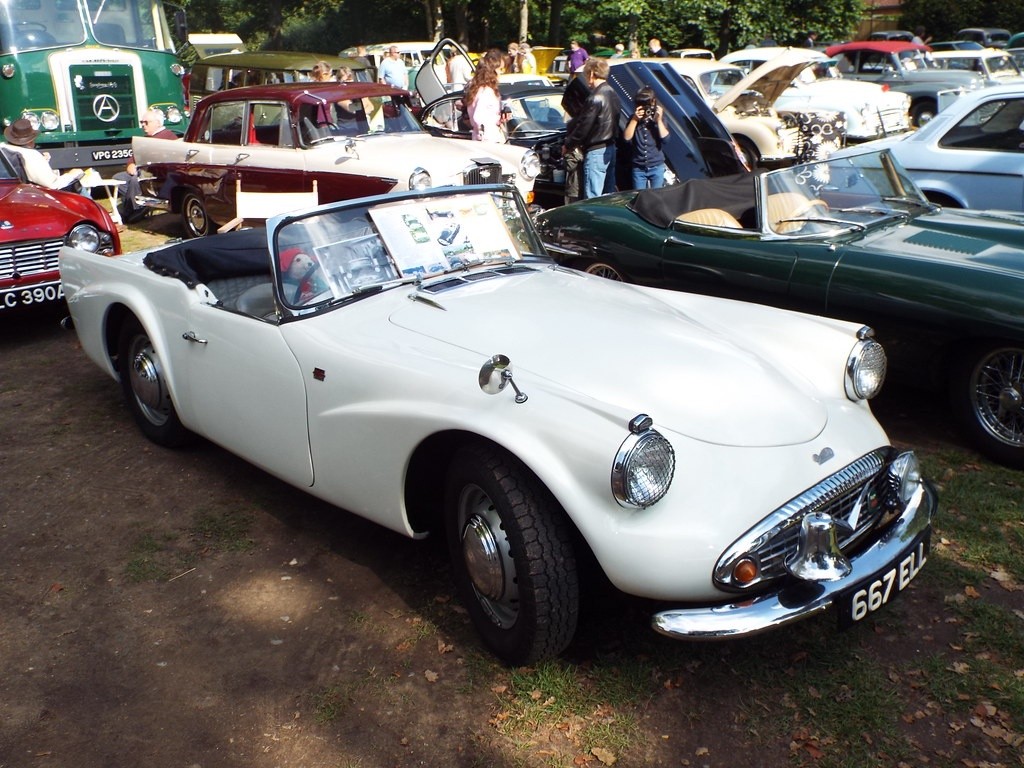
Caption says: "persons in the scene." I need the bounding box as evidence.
[624,87,670,190]
[0,119,92,199]
[562,61,621,205]
[804,32,817,47]
[113,114,178,223]
[379,47,409,90]
[746,33,776,48]
[466,42,536,144]
[611,44,640,58]
[911,26,932,45]
[355,46,374,74]
[312,62,369,131]
[649,39,668,58]
[445,45,473,92]
[218,71,273,91]
[567,41,589,83]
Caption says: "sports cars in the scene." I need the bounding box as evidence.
[54,173,941,671]
[525,144,1024,473]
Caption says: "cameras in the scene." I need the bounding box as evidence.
[641,105,656,116]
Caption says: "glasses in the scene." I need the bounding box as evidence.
[140,119,156,125]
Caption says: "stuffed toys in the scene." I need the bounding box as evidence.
[280,249,317,300]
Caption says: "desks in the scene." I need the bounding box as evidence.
[82,179,127,230]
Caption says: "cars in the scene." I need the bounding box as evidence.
[0,145,126,337]
[123,24,1024,249]
[822,81,1024,214]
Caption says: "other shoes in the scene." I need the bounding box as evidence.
[127,206,149,224]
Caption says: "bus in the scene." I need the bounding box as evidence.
[0,0,189,218]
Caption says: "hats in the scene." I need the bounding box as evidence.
[519,43,530,50]
[3,119,41,146]
[508,42,519,51]
[408,165,430,181]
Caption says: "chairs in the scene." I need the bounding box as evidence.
[677,208,744,229]
[133,163,166,219]
[765,193,818,236]
[96,24,126,46]
[1,149,85,200]
[238,283,302,319]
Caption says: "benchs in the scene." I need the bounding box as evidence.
[212,125,278,145]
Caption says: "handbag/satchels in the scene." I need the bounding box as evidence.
[546,138,586,175]
